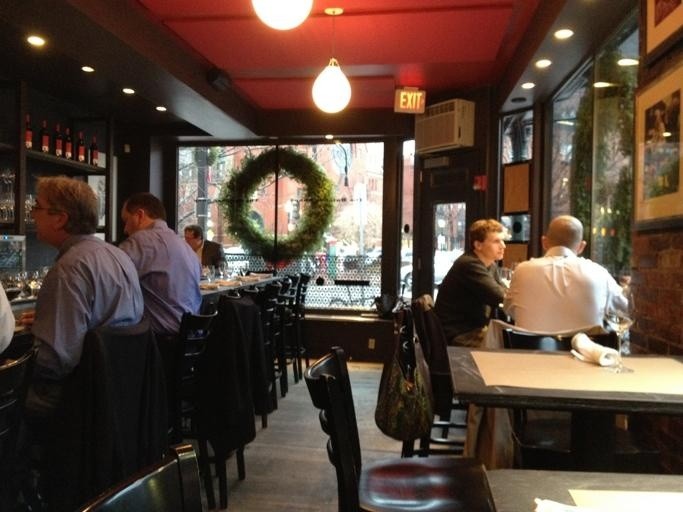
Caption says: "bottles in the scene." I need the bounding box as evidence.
[25,114,99,166]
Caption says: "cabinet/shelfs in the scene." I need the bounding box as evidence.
[0,77,113,244]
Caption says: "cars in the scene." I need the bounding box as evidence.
[218,240,464,291]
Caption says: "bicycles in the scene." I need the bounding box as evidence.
[329,281,378,307]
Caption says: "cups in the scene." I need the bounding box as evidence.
[498,266,512,287]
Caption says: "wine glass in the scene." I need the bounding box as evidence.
[206,261,249,284]
[600,280,637,373]
[0,169,37,223]
[4,266,52,302]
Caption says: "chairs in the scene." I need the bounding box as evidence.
[409,294,470,437]
[1,291,247,512]
[462,318,617,468]
[304,346,498,512]
[392,300,467,458]
[239,272,309,428]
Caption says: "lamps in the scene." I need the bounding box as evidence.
[312,7,352,115]
[252,0,315,31]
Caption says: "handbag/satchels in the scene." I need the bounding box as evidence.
[374,357,436,442]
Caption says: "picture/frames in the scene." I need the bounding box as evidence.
[634,59,683,233]
[498,241,531,268]
[641,0,683,70]
[502,159,531,216]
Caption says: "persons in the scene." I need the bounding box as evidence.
[0,279,29,512]
[19,175,145,415]
[648,108,671,188]
[431,219,510,347]
[183,225,226,270]
[503,214,629,333]
[116,191,203,380]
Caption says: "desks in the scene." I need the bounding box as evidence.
[446,347,682,470]
[487,469,683,512]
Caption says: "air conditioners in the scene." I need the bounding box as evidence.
[414,99,475,155]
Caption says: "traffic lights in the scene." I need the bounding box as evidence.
[289,199,300,221]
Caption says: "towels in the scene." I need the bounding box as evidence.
[570,332,618,366]
